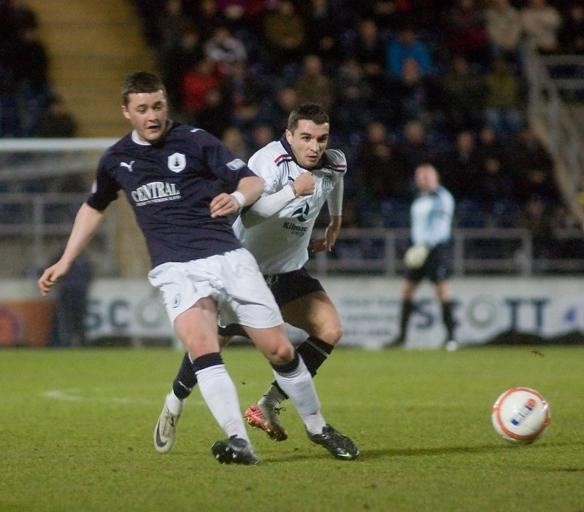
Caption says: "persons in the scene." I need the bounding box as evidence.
[133,2,584,272]
[38,72,360,464]
[153,101,349,453]
[380,164,459,353]
[0,0,93,345]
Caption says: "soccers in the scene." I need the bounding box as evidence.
[491,387,552,442]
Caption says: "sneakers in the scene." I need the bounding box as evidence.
[211,435,258,465]
[304,424,360,461]
[244,404,288,441]
[153,404,180,454]
